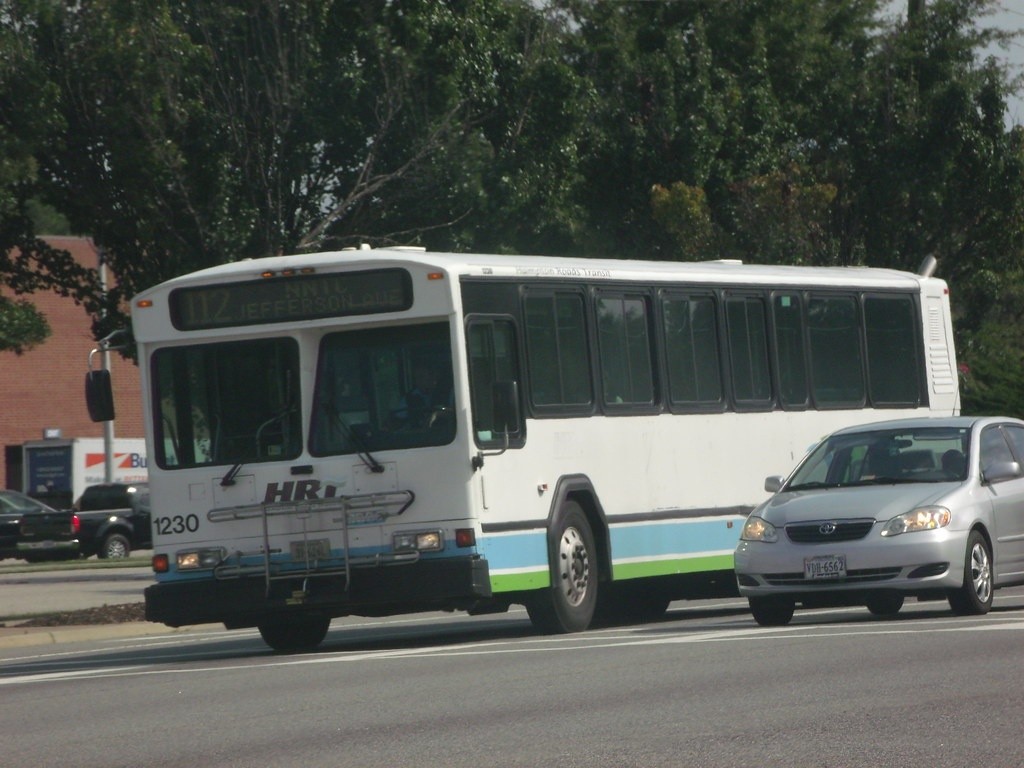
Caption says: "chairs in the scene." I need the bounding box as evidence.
[868,443,905,476]
[941,449,967,479]
[902,449,937,476]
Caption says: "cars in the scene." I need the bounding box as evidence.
[734,416,1024,627]
[0,489,81,566]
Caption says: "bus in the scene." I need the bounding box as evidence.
[84,243,964,660]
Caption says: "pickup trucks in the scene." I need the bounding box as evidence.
[61,482,152,561]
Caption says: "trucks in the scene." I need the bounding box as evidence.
[22,436,212,504]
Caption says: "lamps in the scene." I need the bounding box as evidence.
[43,427,63,440]
